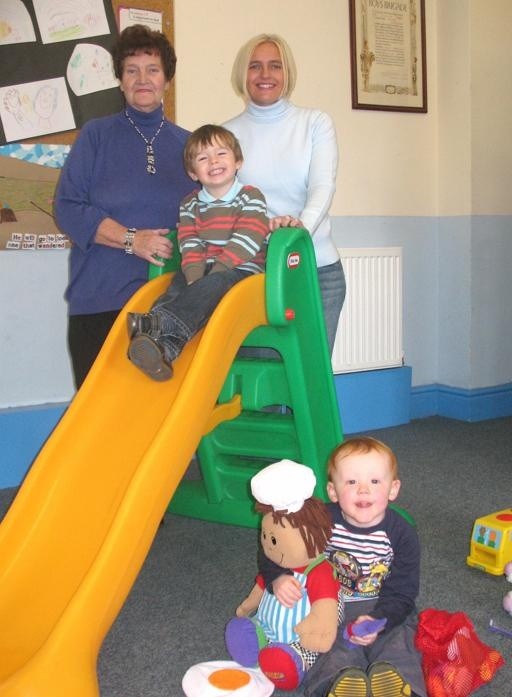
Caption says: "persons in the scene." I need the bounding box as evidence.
[215,32,348,418]
[223,458,341,692]
[52,22,200,528]
[125,123,270,382]
[254,433,428,697]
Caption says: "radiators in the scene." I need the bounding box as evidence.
[330,246,404,378]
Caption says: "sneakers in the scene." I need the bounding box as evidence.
[323,660,413,697]
[125,311,154,336]
[126,333,172,383]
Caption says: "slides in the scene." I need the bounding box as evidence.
[1,272,269,694]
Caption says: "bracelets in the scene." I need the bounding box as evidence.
[124,225,137,255]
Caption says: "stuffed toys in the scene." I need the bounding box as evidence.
[341,616,389,648]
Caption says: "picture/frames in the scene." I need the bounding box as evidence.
[348,0,428,113]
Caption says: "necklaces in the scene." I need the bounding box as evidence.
[124,107,167,176]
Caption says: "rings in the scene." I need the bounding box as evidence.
[153,248,158,257]
